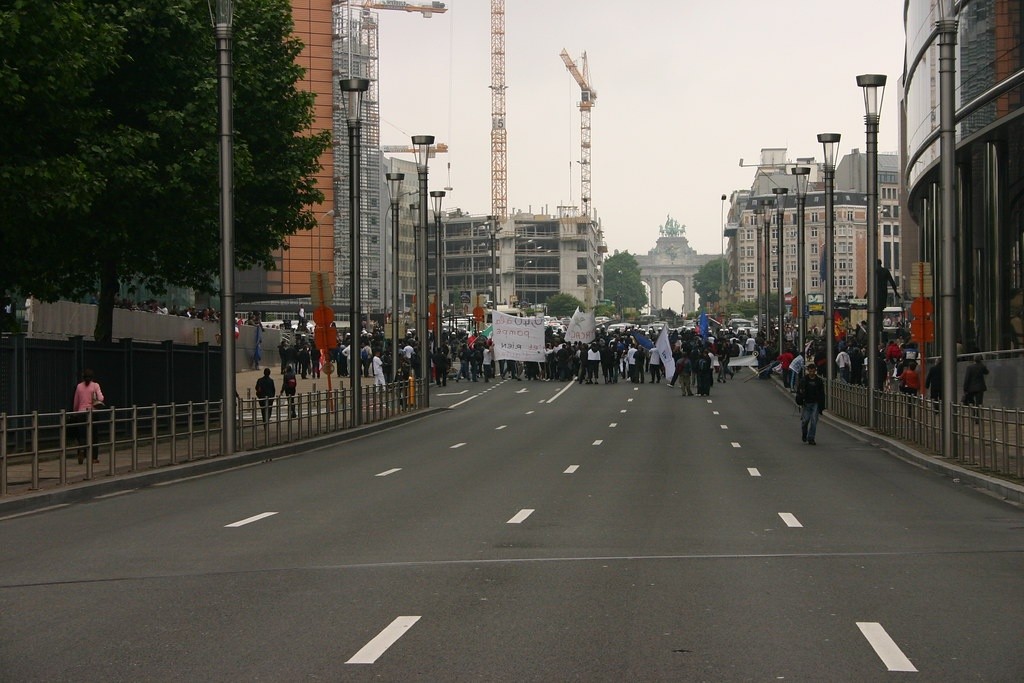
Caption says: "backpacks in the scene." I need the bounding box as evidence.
[699,358,707,371]
[844,362,849,367]
[758,346,766,358]
[362,347,368,359]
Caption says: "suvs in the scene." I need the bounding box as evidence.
[737,325,759,338]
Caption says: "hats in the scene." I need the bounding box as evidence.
[806,364,816,369]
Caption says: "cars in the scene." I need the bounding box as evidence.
[544,314,711,336]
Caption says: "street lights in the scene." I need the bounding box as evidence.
[320,209,335,272]
[817,133,841,406]
[429,191,445,379]
[753,203,772,342]
[207,0,236,453]
[499,234,551,313]
[339,78,369,426]
[856,74,887,426]
[929,0,963,454]
[488,216,498,311]
[721,194,726,325]
[412,135,435,407]
[772,166,811,373]
[384,173,418,391]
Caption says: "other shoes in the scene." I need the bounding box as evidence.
[808,439,816,445]
[802,436,806,442]
[93,459,99,464]
[78,450,84,464]
[667,383,674,388]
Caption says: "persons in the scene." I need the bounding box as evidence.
[898,361,920,418]
[956,338,967,354]
[114,294,266,333]
[394,357,410,414]
[256,368,276,430]
[280,366,297,418]
[278,303,920,396]
[24,293,34,334]
[963,354,989,424]
[863,260,901,309]
[73,369,104,463]
[794,364,825,445]
[925,357,942,415]
[90,291,101,304]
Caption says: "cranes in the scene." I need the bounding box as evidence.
[348,0,448,145]
[561,46,597,215]
[380,137,449,160]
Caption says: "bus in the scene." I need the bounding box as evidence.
[729,318,752,332]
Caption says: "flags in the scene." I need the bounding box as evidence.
[834,309,847,342]
[699,307,708,341]
[655,324,675,381]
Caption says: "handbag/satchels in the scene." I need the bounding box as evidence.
[796,383,803,405]
[92,383,105,409]
[288,379,295,388]
[898,380,904,392]
[960,392,969,406]
